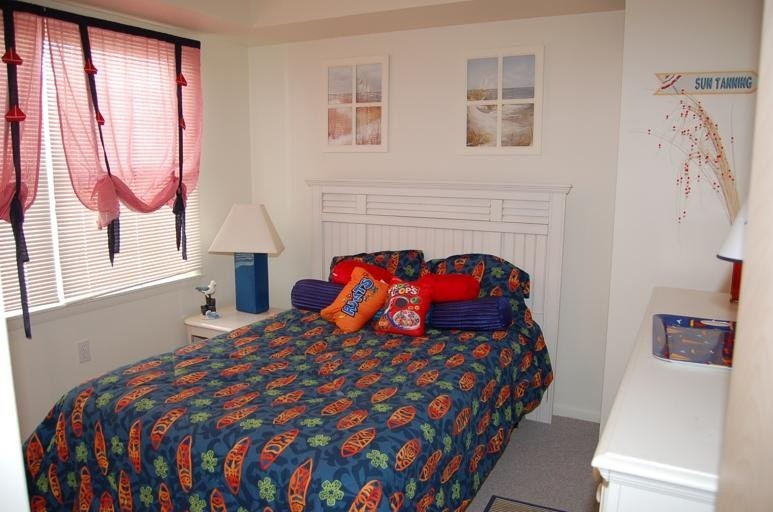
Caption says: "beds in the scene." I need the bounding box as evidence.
[28,176,575,512]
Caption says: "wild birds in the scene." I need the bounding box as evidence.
[195,280,217,298]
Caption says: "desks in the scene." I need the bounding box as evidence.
[588,282,738,512]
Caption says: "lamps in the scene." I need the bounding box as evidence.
[206,202,287,314]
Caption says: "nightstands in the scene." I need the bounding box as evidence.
[183,303,284,347]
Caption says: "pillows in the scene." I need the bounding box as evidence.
[292,248,515,336]
